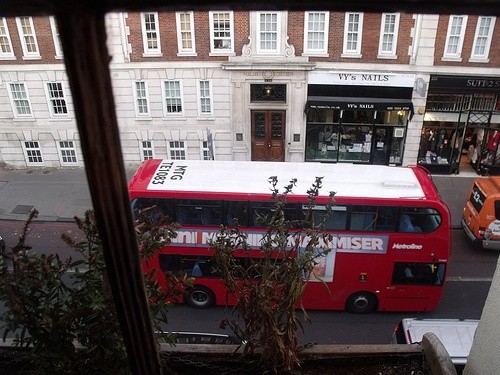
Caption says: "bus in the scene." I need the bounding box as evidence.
[129,158,452,313]
[387,318,484,371]
[462,177,500,250]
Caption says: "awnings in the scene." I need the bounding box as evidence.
[304,96,414,120]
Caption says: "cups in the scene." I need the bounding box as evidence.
[304,254,327,280]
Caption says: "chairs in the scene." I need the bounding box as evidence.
[153,200,263,228]
[401,215,422,234]
